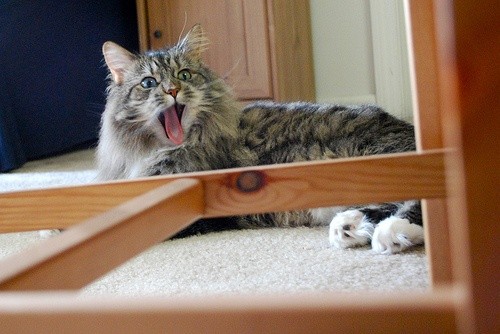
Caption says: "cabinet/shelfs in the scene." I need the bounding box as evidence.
[137,0,317,112]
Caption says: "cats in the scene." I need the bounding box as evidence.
[96,23,426,255]
